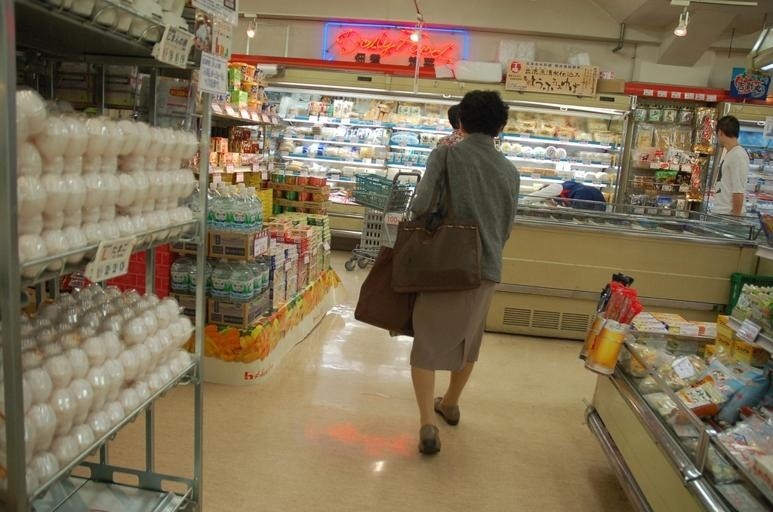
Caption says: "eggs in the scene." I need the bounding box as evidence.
[19,283,194,498]
[16,81,198,281]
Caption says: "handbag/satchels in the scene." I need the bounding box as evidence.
[354,247,415,337]
[390,222,483,293]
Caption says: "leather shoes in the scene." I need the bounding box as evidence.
[434,397,459,424]
[418,424,440,453]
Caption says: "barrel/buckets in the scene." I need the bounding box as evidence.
[170,182,270,300]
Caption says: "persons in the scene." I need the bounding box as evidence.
[410,91,519,453]
[436,105,464,146]
[710,115,750,216]
[523,180,606,210]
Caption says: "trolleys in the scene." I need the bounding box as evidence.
[344,174,424,271]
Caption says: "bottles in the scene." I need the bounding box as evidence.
[188,180,264,234]
[169,249,271,301]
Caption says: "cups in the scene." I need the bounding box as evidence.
[576,311,633,378]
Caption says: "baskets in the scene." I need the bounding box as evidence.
[360,207,383,249]
[355,173,405,210]
